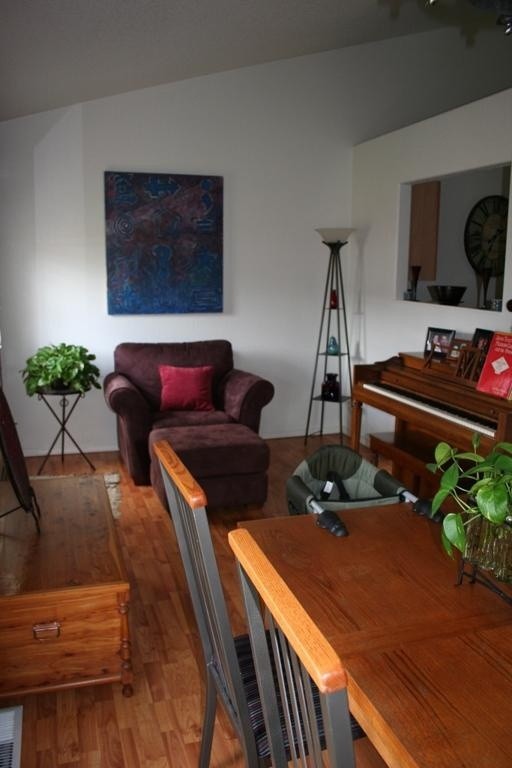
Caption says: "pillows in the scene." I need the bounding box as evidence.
[157,364,216,411]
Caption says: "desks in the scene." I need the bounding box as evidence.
[36,385,96,476]
[236,494,512,768]
[0,475,134,697]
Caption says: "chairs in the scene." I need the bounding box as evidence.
[153,440,366,768]
[104,340,274,485]
[226,527,357,768]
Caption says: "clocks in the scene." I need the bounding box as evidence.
[464,195,508,275]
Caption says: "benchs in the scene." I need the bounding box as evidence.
[369,430,468,512]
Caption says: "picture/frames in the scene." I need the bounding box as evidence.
[447,339,471,362]
[424,327,456,361]
[468,328,494,368]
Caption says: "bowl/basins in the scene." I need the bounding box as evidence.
[427,285,467,305]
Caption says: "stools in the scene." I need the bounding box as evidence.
[148,424,270,520]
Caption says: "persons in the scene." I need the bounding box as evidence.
[470,335,488,377]
[427,334,449,353]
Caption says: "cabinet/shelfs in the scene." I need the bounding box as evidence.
[304,241,352,445]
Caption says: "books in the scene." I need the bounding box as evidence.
[475,331,512,401]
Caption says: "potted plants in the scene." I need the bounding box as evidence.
[426,432,512,583]
[18,343,102,398]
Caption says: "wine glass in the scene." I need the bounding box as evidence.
[411,265,421,301]
